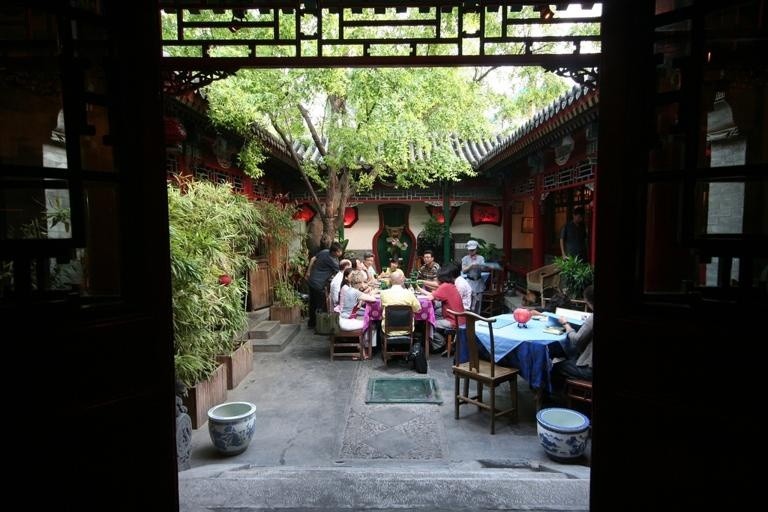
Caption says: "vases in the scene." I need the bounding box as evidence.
[208,402,256,454]
[536,407,590,461]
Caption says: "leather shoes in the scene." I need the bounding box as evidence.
[352,354,369,360]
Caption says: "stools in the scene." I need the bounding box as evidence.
[444,329,456,358]
[562,377,593,408]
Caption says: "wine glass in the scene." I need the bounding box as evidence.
[382,266,387,273]
[404,270,424,295]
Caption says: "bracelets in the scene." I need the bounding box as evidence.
[562,322,568,325]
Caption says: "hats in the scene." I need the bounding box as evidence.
[466,240,478,250]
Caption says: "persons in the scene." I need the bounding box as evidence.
[549,284,594,397]
[559,207,588,265]
[303,239,486,360]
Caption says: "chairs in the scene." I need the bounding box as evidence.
[325,287,334,317]
[330,293,363,362]
[526,263,562,310]
[379,305,413,368]
[482,270,504,317]
[445,309,520,435]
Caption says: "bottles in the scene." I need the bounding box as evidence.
[381,278,389,290]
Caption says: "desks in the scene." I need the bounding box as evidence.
[459,312,585,419]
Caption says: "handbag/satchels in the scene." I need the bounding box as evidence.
[314,309,341,336]
[406,342,427,374]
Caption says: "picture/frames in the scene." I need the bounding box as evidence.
[512,200,524,214]
[521,216,534,233]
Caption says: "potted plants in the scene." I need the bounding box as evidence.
[167,168,267,430]
[270,201,302,324]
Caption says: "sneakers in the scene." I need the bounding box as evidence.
[441,342,453,357]
